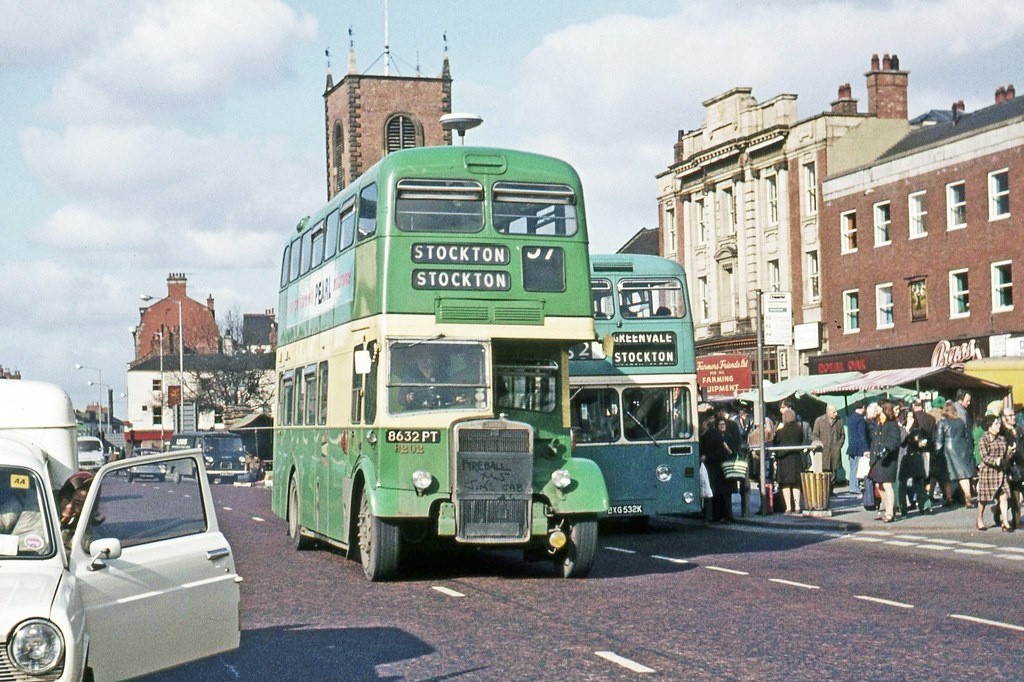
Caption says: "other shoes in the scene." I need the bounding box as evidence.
[899,514,907,519]
[785,510,792,514]
[908,503,916,512]
[965,502,977,509]
[883,516,894,524]
[991,505,1000,525]
[721,518,726,524]
[943,497,955,508]
[795,507,800,512]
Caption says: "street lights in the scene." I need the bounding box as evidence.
[140,293,184,433]
[128,327,164,453]
[88,381,109,434]
[76,364,101,440]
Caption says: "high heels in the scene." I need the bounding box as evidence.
[976,521,986,530]
[1001,523,1014,533]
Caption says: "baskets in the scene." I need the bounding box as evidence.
[722,452,747,481]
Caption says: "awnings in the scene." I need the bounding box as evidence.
[811,367,1014,487]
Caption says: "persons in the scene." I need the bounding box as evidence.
[856,388,974,511]
[846,400,871,494]
[991,408,1024,527]
[811,404,845,497]
[976,415,1017,532]
[774,410,805,514]
[699,418,738,526]
[893,410,935,519]
[933,399,978,509]
[0,472,105,560]
[698,398,812,518]
[398,351,466,412]
[869,401,901,523]
[114,446,127,474]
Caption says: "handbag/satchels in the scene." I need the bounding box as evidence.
[856,457,871,480]
[1009,459,1023,484]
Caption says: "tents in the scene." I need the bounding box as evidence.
[713,371,923,487]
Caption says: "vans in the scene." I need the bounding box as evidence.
[167,431,246,484]
[77,436,105,472]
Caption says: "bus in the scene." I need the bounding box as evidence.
[495,253,708,524]
[271,147,609,583]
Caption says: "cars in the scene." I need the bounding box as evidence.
[0,436,244,682]
[126,448,167,482]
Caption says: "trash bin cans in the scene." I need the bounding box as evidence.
[249,462,265,481]
[800,471,832,511]
